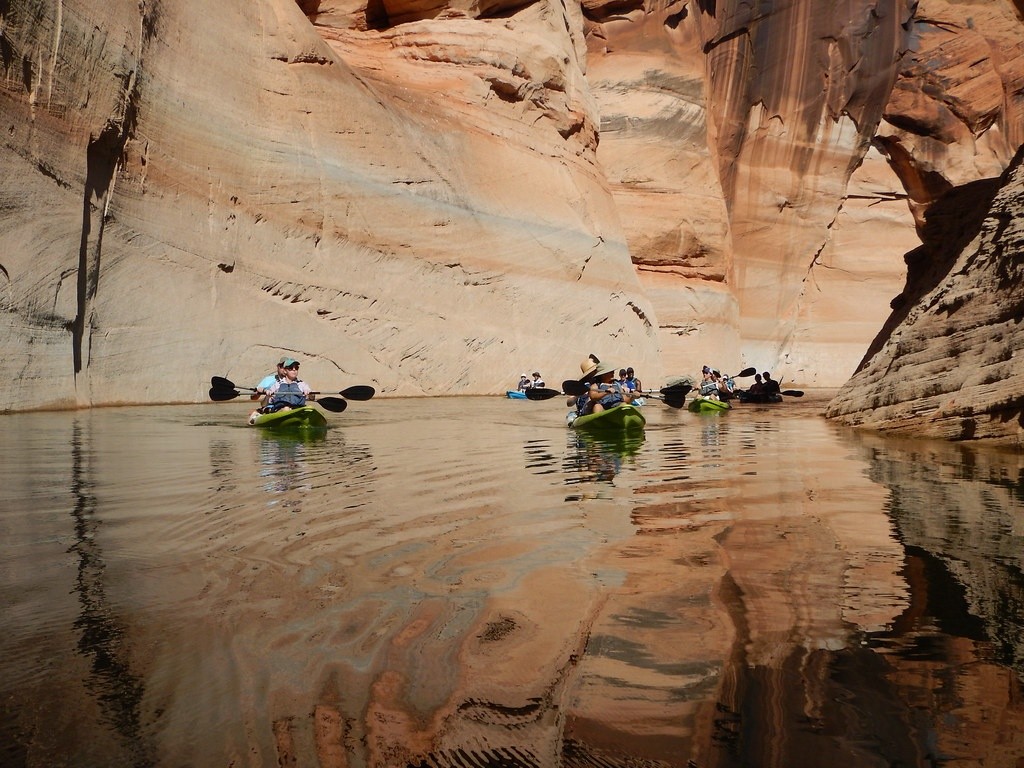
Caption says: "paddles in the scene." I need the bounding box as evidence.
[734,390,804,397]
[524,384,692,402]
[685,367,756,396]
[208,384,376,402]
[210,375,348,414]
[562,379,686,410]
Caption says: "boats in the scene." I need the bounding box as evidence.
[506,390,528,399]
[741,393,783,404]
[525,388,559,401]
[687,398,730,412]
[246,404,328,429]
[632,397,645,406]
[564,404,647,432]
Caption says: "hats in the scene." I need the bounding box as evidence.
[619,369,627,375]
[627,368,634,373]
[702,368,713,376]
[712,368,721,377]
[521,374,526,377]
[532,372,541,378]
[283,358,301,368]
[723,376,727,379]
[279,357,289,363]
[579,359,601,381]
[593,361,620,377]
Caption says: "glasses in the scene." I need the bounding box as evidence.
[285,366,299,371]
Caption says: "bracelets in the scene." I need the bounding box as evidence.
[309,398,315,401]
[263,388,266,393]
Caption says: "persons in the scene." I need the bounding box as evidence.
[249,356,316,415]
[566,358,640,417]
[748,372,780,394]
[518,372,545,393]
[618,367,642,393]
[695,366,737,401]
